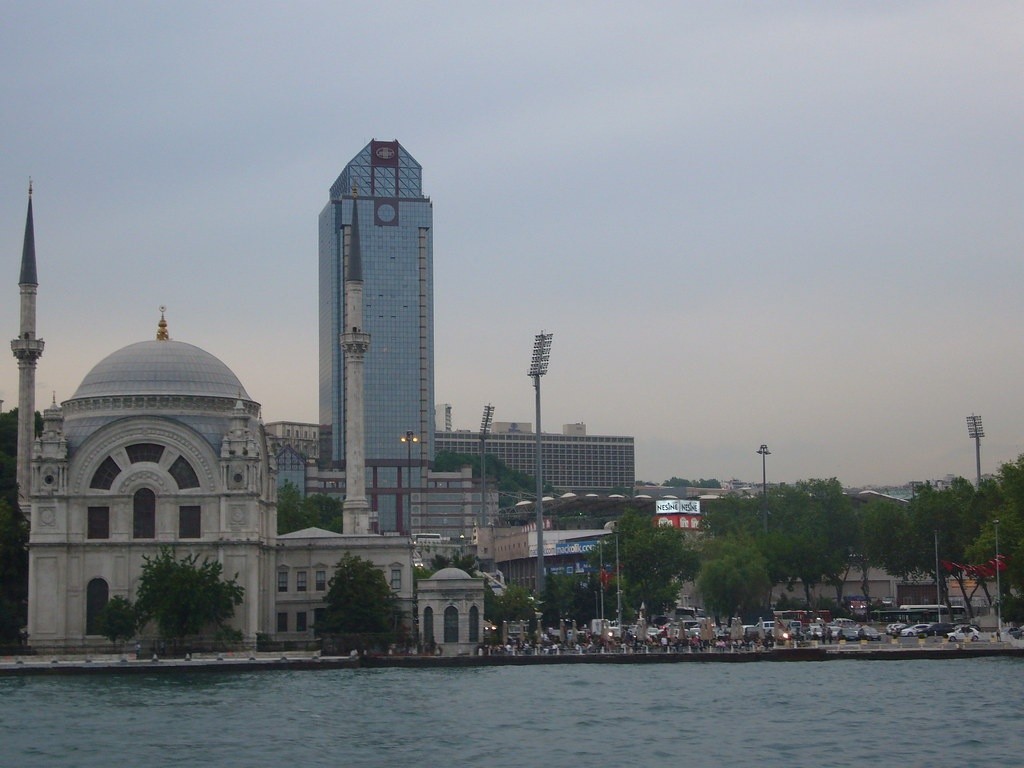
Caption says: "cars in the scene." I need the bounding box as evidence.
[889,624,900,635]
[814,619,826,627]
[892,625,906,635]
[858,627,864,638]
[809,626,823,639]
[808,623,820,629]
[917,623,952,636]
[838,628,859,641]
[886,624,893,634]
[863,628,881,641]
[1003,626,1024,639]
[540,620,755,642]
[952,624,979,634]
[944,627,979,642]
[827,627,841,638]
[900,624,933,637]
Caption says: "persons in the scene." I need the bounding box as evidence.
[996,629,1001,642]
[491,624,899,657]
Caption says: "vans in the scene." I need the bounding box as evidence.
[756,621,776,633]
[837,618,857,628]
[789,621,802,629]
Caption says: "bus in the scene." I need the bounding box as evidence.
[900,605,966,620]
[675,606,706,620]
[869,609,932,626]
[412,534,442,544]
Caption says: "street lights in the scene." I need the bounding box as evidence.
[967,413,986,482]
[859,489,942,625]
[480,403,493,523]
[993,520,1001,633]
[528,329,553,596]
[402,431,418,535]
[757,444,771,535]
